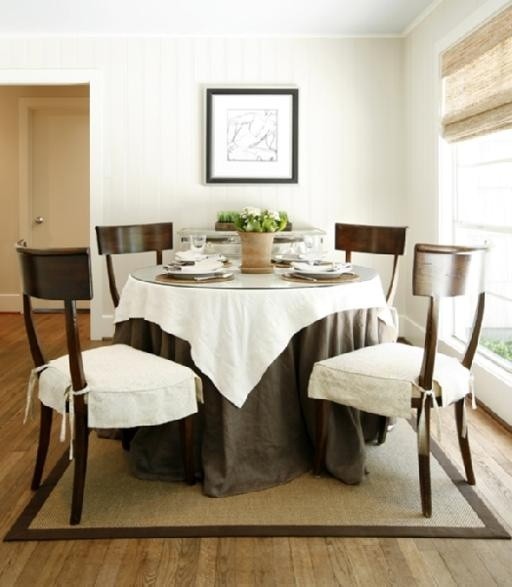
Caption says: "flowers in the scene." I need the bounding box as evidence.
[234,208,289,234]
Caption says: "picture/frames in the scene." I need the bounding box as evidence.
[205,85,300,185]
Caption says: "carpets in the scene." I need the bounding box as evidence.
[2,411,512,544]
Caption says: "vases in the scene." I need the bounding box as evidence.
[238,231,277,275]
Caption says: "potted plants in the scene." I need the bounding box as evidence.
[214,210,240,232]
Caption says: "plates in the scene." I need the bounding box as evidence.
[159,262,224,279]
[175,254,228,264]
[275,253,345,264]
[295,266,354,277]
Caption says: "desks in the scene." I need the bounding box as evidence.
[178,226,326,242]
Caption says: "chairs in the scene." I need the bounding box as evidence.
[14,238,205,525]
[334,222,411,307]
[95,223,174,309]
[306,239,491,520]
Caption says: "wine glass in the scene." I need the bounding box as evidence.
[304,235,315,258]
[190,235,207,259]
